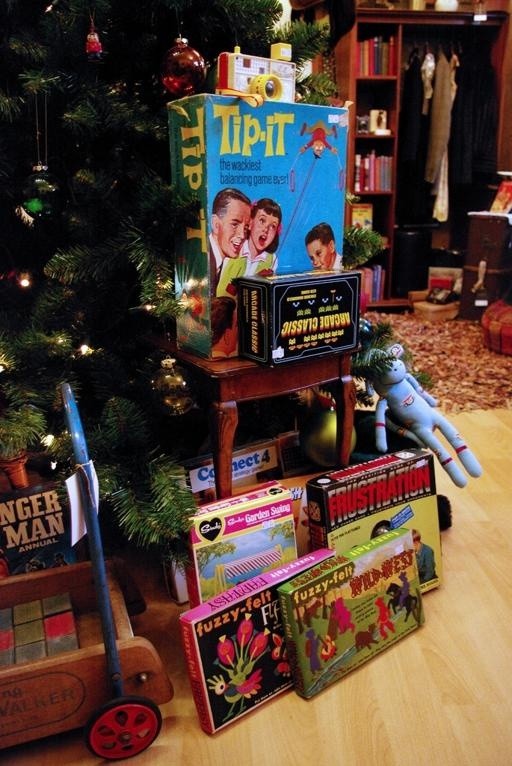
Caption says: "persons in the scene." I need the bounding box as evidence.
[209,293,239,359]
[304,222,345,270]
[410,528,438,587]
[209,187,250,298]
[217,196,284,312]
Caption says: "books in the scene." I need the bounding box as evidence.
[350,32,393,304]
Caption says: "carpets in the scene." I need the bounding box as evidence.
[355,311,511,414]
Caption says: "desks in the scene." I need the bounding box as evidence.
[159,342,357,501]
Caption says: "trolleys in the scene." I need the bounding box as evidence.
[1,376,176,762]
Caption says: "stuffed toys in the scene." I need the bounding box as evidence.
[363,342,483,490]
